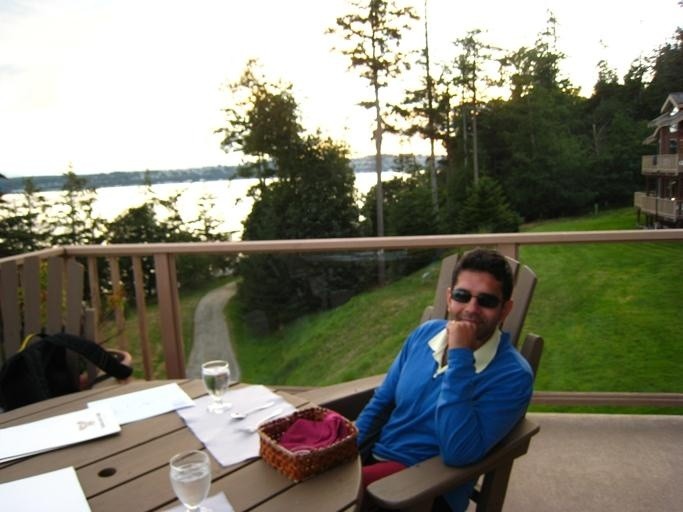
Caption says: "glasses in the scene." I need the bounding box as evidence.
[450,288,502,308]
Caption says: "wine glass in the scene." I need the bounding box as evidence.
[200,358,231,419]
[167,449,211,512]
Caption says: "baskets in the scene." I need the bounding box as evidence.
[257,406,360,484]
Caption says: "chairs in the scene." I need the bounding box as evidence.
[0,255,96,391]
[294,248,544,512]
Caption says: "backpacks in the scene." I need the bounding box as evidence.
[0,331,133,413]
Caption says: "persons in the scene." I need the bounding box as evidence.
[351,248,532,512]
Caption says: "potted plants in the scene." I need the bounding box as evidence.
[17,263,131,388]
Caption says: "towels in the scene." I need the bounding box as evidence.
[280,412,341,454]
